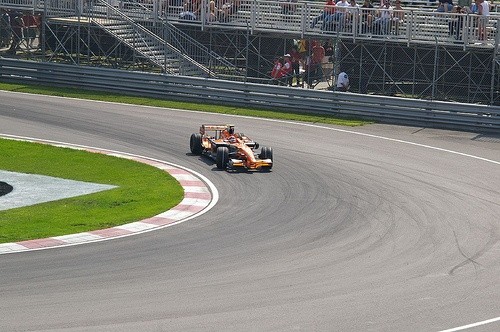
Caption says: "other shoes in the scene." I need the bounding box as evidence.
[297,83,299,85]
[289,84,292,87]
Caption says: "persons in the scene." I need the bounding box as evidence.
[229,136,236,143]
[282,53,292,86]
[433,0,489,45]
[179,0,241,22]
[288,46,301,86]
[0,11,42,51]
[272,57,282,84]
[337,67,349,92]
[303,40,325,86]
[310,0,405,39]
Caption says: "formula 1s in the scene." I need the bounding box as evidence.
[190,124,273,169]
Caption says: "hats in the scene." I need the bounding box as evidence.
[284,53,290,56]
[274,57,280,60]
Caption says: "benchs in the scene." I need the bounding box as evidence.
[90,2,499,46]
